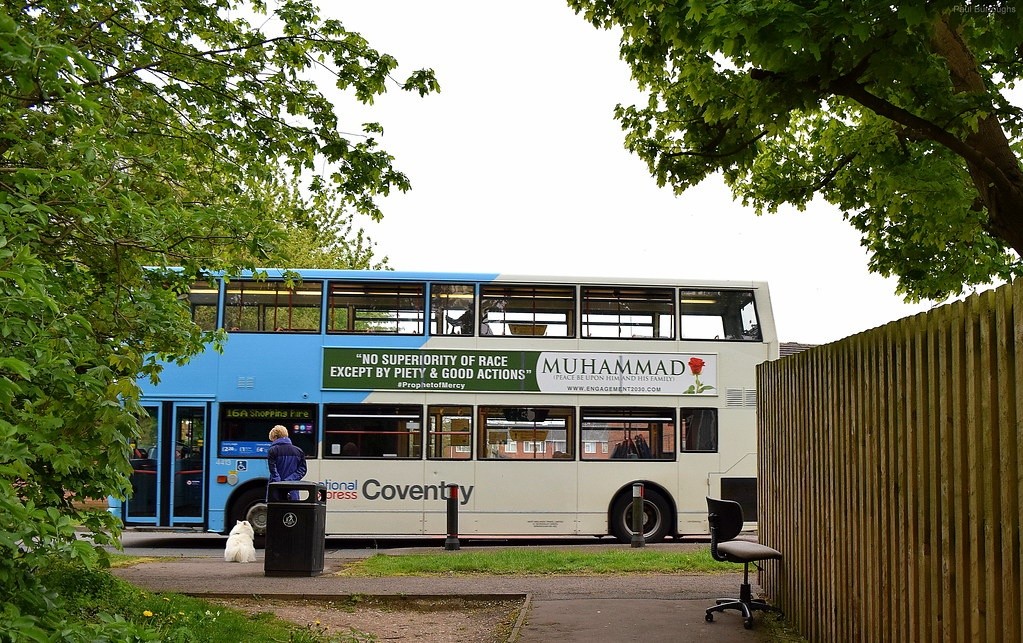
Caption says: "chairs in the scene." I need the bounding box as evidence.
[608,433,653,460]
[705,496,786,629]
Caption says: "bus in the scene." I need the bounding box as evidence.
[103,267,779,549]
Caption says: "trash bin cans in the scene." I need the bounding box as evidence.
[266,481,327,577]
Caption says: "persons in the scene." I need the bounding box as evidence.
[265,425,308,502]
[343,442,357,456]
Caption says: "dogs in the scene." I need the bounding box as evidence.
[224,519,257,563]
